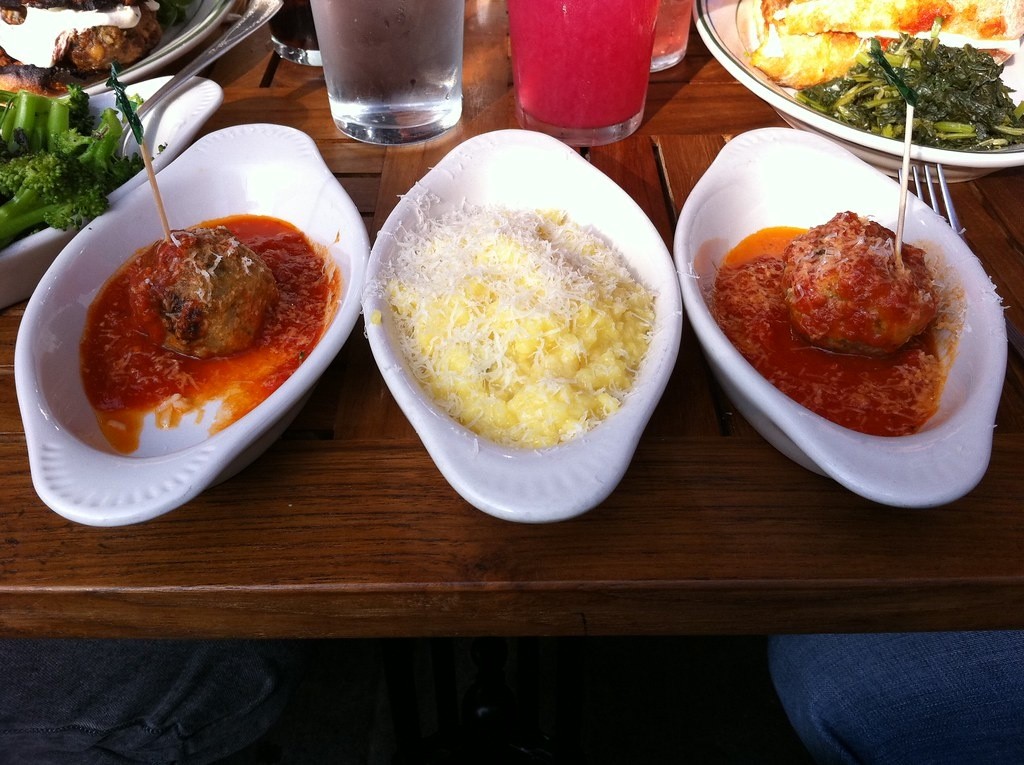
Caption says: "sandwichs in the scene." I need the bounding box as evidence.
[747,0,1024,89]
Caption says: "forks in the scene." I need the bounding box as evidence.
[897,164,1024,360]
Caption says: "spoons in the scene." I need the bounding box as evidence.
[114,0,283,161]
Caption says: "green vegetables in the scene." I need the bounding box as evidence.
[796,15,1024,153]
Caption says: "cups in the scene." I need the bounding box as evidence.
[508,0,658,146]
[310,1,466,146]
[268,0,322,66]
[650,0,692,72]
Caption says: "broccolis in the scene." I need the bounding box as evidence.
[0,84,165,252]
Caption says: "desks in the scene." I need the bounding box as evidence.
[0,0,1024,638]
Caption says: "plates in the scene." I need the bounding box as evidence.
[0,75,224,308]
[15,124,370,526]
[673,127,1008,507]
[0,0,234,99]
[366,129,682,523]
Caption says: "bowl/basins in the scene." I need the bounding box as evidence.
[693,0,1024,183]
